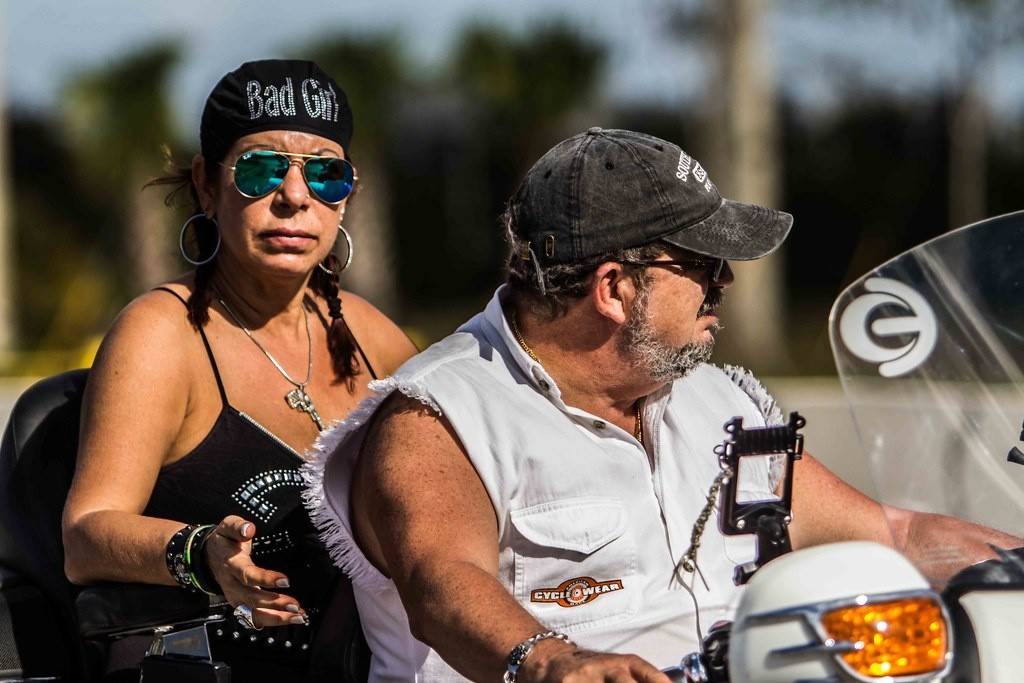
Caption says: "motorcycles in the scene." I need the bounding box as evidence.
[0,209,1023,683]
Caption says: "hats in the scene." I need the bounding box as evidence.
[503,127,796,298]
[201,60,353,185]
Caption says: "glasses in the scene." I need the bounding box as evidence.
[219,149,359,205]
[568,250,726,282]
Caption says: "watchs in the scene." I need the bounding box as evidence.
[500,628,578,683]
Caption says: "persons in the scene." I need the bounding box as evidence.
[349,132,1024,683]
[58,59,431,683]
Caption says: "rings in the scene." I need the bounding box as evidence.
[232,603,265,631]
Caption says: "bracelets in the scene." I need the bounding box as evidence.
[165,524,224,598]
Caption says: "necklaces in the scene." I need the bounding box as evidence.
[507,315,645,452]
[198,267,325,432]
